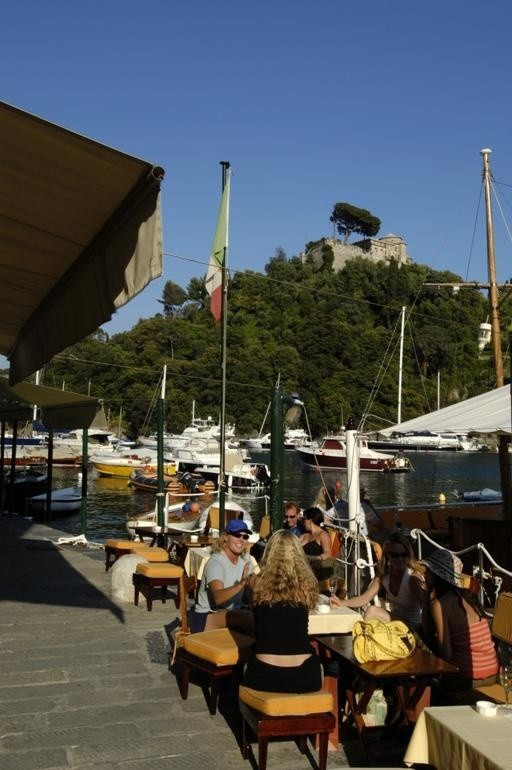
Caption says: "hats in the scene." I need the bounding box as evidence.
[421,548,464,586]
[225,520,253,534]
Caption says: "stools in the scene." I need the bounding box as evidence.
[176,627,255,717]
[132,562,184,611]
[131,545,170,562]
[236,684,337,769]
[103,538,145,574]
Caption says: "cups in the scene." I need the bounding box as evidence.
[190,535,198,543]
[316,605,330,613]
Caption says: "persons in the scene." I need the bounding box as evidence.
[419,549,501,710]
[280,480,388,582]
[329,532,432,647]
[189,518,263,635]
[233,528,324,693]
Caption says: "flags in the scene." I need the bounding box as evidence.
[204,171,231,325]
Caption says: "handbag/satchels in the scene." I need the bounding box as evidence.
[354,619,415,664]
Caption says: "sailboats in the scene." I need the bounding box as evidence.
[353,305,473,451]
[329,146,512,619]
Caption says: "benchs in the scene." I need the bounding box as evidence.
[244,513,342,592]
[169,505,245,551]
[474,590,511,705]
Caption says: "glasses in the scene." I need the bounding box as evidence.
[231,534,249,540]
[283,515,296,519]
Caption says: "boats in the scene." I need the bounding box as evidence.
[126,466,216,496]
[1,367,252,478]
[452,486,502,504]
[124,497,201,542]
[295,408,410,473]
[192,462,272,496]
[238,370,313,454]
[200,497,260,542]
[311,486,377,529]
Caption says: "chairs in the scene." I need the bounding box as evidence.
[170,569,199,668]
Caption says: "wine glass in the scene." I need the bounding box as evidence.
[498,665,512,715]
[328,577,339,606]
